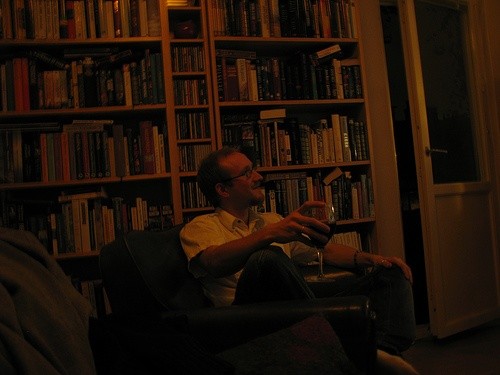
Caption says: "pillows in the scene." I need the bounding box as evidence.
[218,313,357,375]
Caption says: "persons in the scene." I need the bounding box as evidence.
[178,146,422,375]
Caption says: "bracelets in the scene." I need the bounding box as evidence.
[352,248,364,266]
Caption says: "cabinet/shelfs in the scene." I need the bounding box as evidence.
[0,0,378,314]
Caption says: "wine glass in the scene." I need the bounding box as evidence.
[305,206,337,282]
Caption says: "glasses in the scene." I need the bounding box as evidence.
[218,164,258,187]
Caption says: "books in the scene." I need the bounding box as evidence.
[1,188,177,318]
[0,0,160,39]
[212,0,359,38]
[1,45,165,113]
[1,117,167,184]
[171,42,365,106]
[181,177,374,274]
[176,108,370,173]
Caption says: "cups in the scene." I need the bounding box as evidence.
[172,24,198,39]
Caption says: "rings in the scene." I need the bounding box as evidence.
[380,259,387,264]
[299,223,306,231]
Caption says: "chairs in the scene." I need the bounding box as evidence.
[99,222,378,375]
